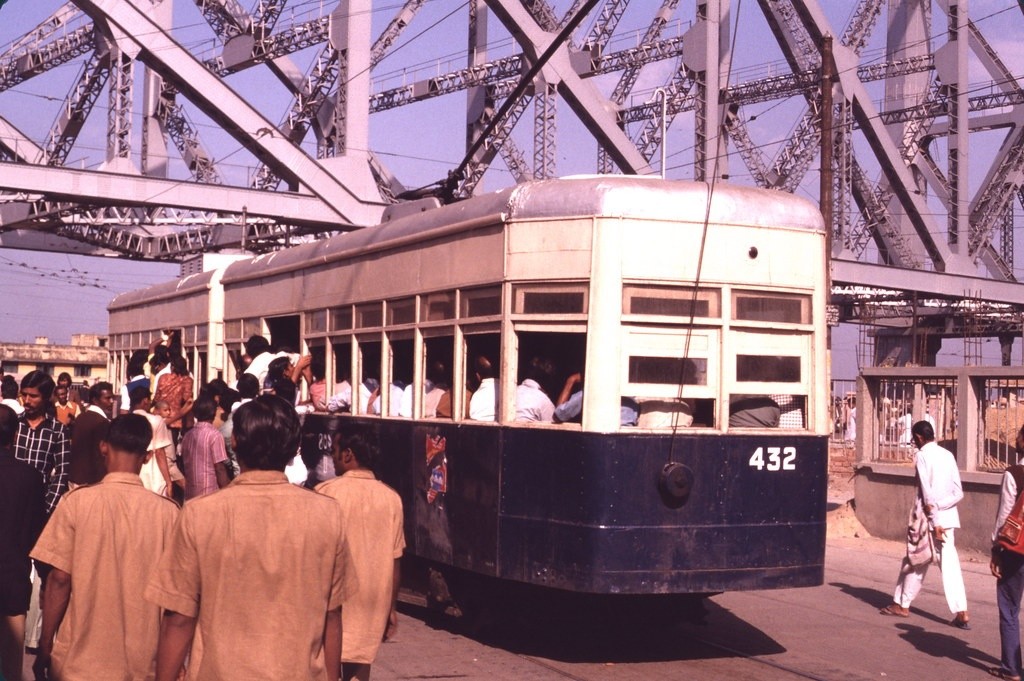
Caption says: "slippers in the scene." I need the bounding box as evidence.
[987,667,1021,681]
[953,617,971,630]
[880,606,908,617]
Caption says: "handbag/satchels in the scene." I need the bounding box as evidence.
[907,504,936,569]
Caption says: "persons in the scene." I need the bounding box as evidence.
[986,424,1024,681]
[0,333,784,681]
[878,421,972,630]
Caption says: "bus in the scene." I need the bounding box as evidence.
[107,174,828,618]
